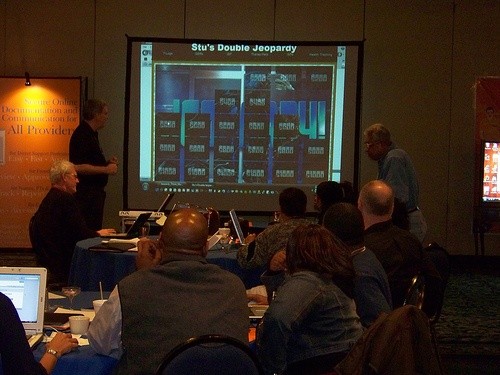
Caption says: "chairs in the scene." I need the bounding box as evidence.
[154,334,264,375]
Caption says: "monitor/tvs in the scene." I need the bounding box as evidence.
[479,140,500,208]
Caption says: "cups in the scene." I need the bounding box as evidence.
[93,300,108,315]
[69,316,90,334]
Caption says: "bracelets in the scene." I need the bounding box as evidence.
[46,348,59,361]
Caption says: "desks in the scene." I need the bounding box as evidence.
[33,290,118,375]
[69,237,265,290]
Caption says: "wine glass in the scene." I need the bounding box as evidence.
[62,286,81,308]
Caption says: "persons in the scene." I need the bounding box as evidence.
[88,209,249,375]
[363,123,428,242]
[30,160,117,290]
[247,225,365,375]
[237,180,360,270]
[68,98,119,239]
[0,293,79,375]
[325,203,393,326]
[356,181,425,310]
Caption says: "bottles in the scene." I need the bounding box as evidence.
[219,222,231,244]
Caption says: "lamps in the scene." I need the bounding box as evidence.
[24,71,31,85]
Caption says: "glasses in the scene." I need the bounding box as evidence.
[364,142,380,147]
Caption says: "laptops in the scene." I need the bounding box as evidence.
[0,267,47,352]
[247,307,268,323]
[101,213,152,239]
[157,193,175,212]
[230,209,248,245]
